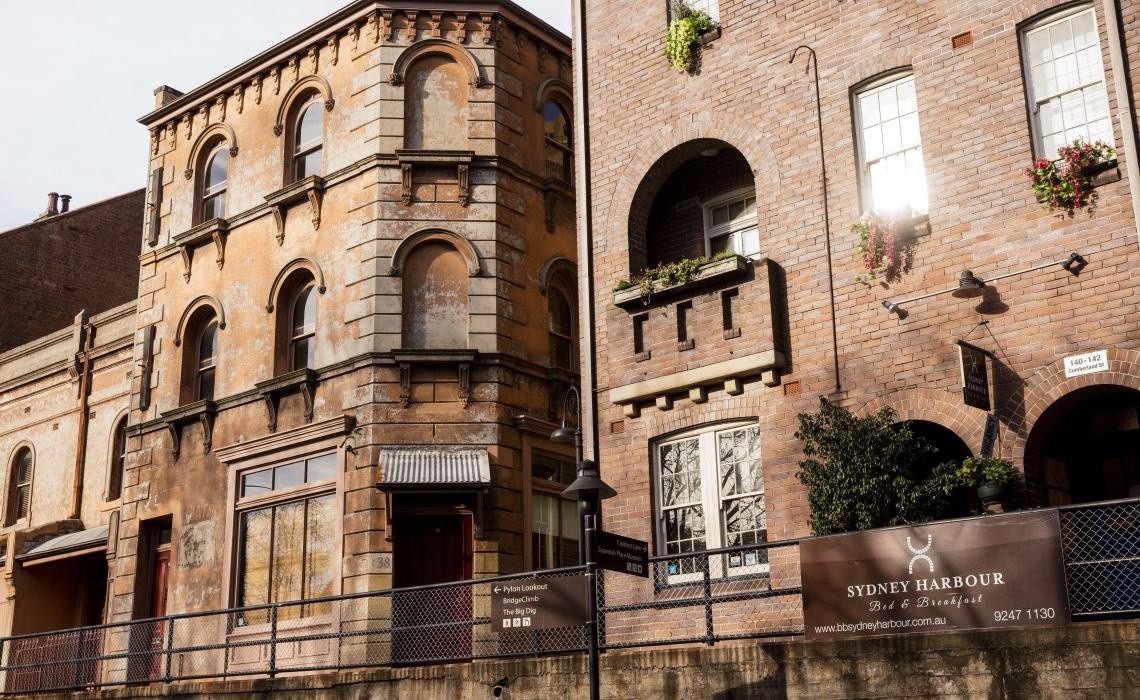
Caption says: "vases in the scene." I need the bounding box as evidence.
[1052,156,1099,175]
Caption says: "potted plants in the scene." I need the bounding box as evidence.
[957,456,1021,504]
[611,251,749,306]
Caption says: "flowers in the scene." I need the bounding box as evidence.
[850,203,916,282]
[1024,139,1115,216]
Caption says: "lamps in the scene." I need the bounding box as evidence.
[1060,253,1079,271]
[881,299,899,314]
[952,270,983,299]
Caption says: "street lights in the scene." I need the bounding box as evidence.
[549,385,589,656]
[558,458,619,699]
